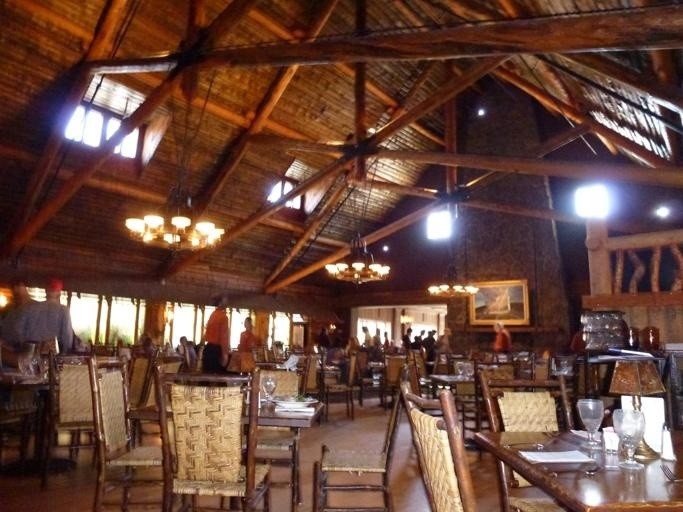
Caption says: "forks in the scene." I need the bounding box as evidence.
[659,462,683,483]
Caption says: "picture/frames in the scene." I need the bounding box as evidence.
[468,278,531,327]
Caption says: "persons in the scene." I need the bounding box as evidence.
[7,277,73,445]
[1,282,40,344]
[176,334,197,376]
[362,326,453,361]
[138,338,156,372]
[492,322,513,363]
[197,294,230,389]
[317,326,359,367]
[237,317,257,356]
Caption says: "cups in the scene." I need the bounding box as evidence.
[627,325,660,352]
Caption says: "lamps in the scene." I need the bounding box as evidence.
[124,70,227,250]
[428,205,482,299]
[325,153,391,285]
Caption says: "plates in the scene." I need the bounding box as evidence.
[273,396,319,408]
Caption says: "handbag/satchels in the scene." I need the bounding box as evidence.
[227,351,255,372]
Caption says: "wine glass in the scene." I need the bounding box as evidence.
[452,351,576,382]
[577,398,604,450]
[18,356,81,381]
[612,409,646,470]
[262,372,276,405]
